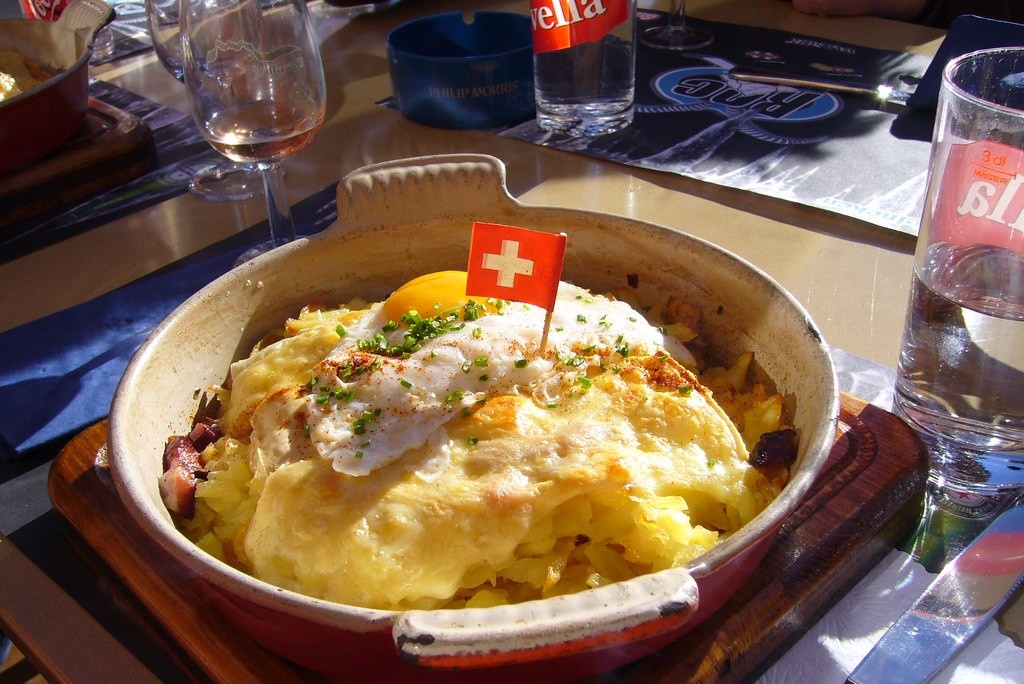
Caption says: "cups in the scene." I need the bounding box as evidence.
[891,46,1023,491]
[528,0,635,137]
[19,0,115,64]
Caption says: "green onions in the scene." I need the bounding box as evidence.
[301,288,696,460]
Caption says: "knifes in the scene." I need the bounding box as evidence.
[845,492,1024,684]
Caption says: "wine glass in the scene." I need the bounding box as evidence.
[142,0,285,201]
[179,1,326,267]
[636,0,717,48]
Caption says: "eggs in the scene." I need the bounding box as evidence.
[307,270,698,477]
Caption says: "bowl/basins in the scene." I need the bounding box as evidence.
[0,0,116,172]
[105,154,837,684]
